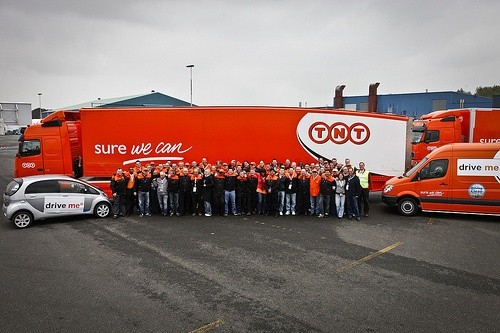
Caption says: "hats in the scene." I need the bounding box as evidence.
[324,161,328,164]
[185,163,189,165]
[222,163,227,166]
[333,169,338,171]
[150,162,155,165]
[144,168,148,171]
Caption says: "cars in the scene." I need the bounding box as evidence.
[5,175,111,228]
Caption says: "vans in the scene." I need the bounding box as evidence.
[382,143,500,217]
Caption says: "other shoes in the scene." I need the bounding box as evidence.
[291,210,295,215]
[139,213,144,218]
[119,212,126,216]
[324,212,328,215]
[146,213,151,216]
[126,211,135,216]
[316,213,319,217]
[304,211,308,215]
[322,213,328,218]
[285,210,290,215]
[252,208,279,217]
[113,216,117,219]
[296,210,301,216]
[352,213,355,216]
[356,216,360,221]
[160,212,251,217]
[279,211,283,216]
[348,215,352,220]
[309,213,314,216]
[365,212,369,216]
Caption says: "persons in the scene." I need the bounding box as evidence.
[110,157,371,220]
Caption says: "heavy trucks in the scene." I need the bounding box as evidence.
[15,103,409,202]
[410,106,498,169]
